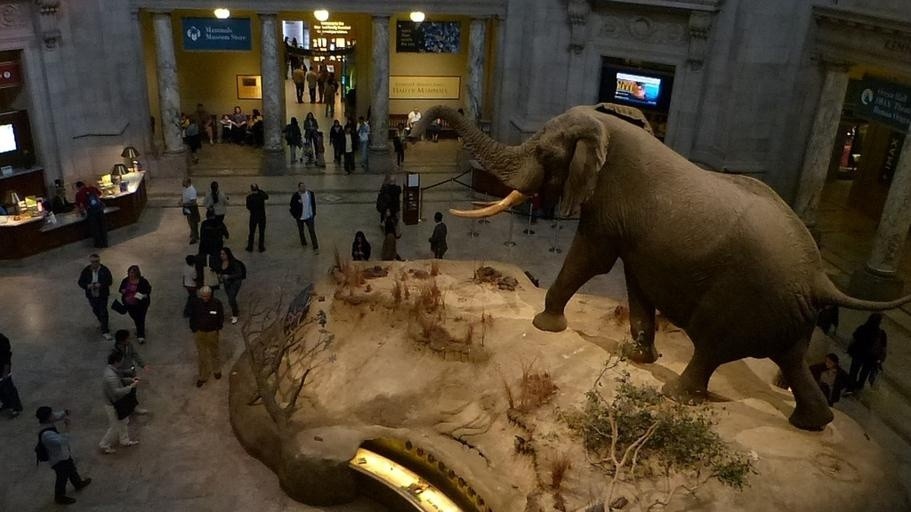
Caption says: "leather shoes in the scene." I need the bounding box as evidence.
[76,478,91,490]
[198,373,221,386]
[55,496,75,504]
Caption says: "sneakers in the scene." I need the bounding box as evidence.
[230,317,238,323]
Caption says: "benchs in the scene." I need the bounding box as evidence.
[222,114,263,144]
[185,114,218,143]
[389,113,421,141]
[425,117,454,140]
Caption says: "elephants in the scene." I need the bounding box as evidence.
[406,102,911,432]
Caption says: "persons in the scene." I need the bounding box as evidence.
[811,302,889,407]
[1,32,466,504]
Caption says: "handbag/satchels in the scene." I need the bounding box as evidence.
[111,299,127,315]
[114,388,138,420]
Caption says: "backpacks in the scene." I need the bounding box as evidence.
[35,427,58,463]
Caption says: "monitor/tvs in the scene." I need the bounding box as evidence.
[0,122,23,159]
[611,71,663,109]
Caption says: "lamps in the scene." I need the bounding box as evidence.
[121,146,140,168]
[111,164,130,181]
[2,190,21,215]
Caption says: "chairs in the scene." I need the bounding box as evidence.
[42,198,56,217]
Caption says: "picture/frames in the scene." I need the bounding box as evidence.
[389,74,461,100]
[236,74,263,100]
